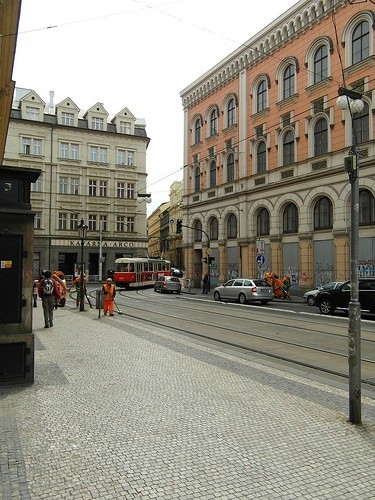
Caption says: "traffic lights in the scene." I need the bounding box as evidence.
[203,257,207,264]
[177,219,183,233]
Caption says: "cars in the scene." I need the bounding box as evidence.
[303,282,351,306]
[213,278,274,304]
[172,269,183,278]
[154,276,181,294]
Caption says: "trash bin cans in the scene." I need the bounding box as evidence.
[96,289,105,309]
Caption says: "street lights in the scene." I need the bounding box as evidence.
[77,218,88,311]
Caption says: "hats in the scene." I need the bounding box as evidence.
[107,278,112,281]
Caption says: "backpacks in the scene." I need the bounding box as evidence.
[43,280,54,296]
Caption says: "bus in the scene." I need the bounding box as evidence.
[114,256,171,289]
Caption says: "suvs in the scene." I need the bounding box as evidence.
[314,277,375,314]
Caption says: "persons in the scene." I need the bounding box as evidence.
[69,273,87,307]
[266,271,294,298]
[201,272,210,294]
[102,278,117,316]
[33,271,69,328]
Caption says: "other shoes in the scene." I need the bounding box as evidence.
[50,324,53,327]
[44,326,49,328]
[104,313,106,315]
[110,314,114,316]
